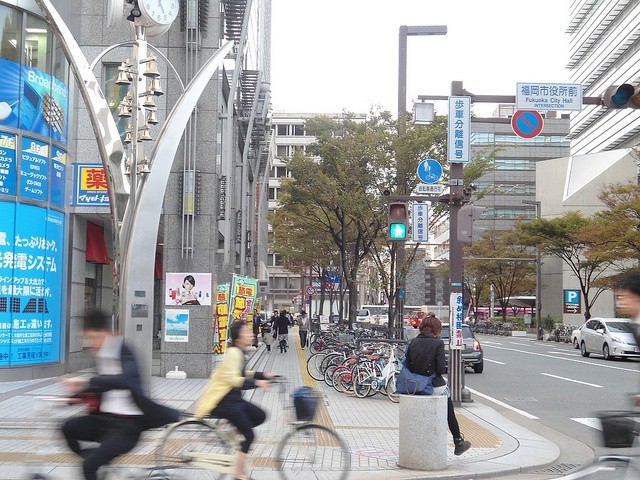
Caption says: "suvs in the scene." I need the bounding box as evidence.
[329,315,339,323]
[440,324,484,374]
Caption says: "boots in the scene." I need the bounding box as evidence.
[454,433,471,455]
[284,343,286,352]
[280,343,283,353]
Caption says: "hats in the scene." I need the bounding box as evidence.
[300,311,307,314]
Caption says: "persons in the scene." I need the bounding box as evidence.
[268,311,280,340]
[401,314,480,456]
[614,275,639,334]
[253,307,262,348]
[175,276,200,304]
[276,312,291,352]
[198,314,274,480]
[57,307,179,480]
[284,309,293,324]
[297,311,311,350]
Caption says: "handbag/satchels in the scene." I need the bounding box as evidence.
[307,334,309,347]
[397,338,436,394]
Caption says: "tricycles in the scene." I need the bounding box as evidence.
[409,311,425,328]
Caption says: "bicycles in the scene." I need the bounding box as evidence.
[17,387,189,475]
[157,374,352,479]
[307,320,408,402]
[553,322,577,343]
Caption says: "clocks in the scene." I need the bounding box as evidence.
[138,0,181,26]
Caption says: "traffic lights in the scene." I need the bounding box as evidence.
[389,203,408,241]
[605,84,640,108]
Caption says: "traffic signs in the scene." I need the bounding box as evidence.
[563,289,581,314]
[325,271,341,282]
[418,159,444,184]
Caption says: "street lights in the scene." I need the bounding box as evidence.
[413,81,474,404]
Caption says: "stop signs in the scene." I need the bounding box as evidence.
[511,109,544,139]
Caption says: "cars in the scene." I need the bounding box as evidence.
[577,318,639,360]
[571,322,583,350]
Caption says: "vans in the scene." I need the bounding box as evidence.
[356,310,371,323]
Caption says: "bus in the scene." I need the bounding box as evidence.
[469,307,536,324]
[360,305,428,328]
